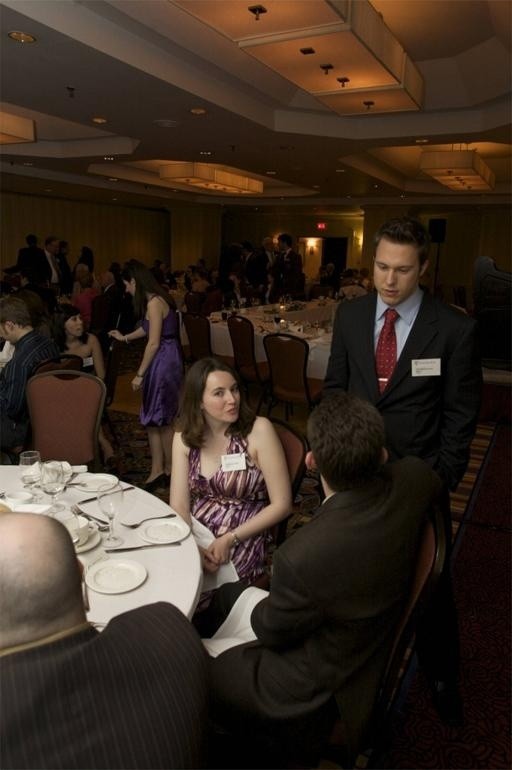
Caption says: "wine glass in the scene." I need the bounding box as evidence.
[40,460,66,513]
[18,451,44,500]
[96,484,125,548]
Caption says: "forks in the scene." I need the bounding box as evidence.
[120,514,177,529]
[70,504,109,524]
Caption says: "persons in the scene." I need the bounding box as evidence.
[324,219,485,724]
[317,261,371,300]
[167,356,294,634]
[1,228,305,468]
[107,259,185,492]
[1,510,211,770]
[193,391,450,770]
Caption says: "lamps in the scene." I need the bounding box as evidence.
[0,109,38,153]
[158,157,263,199]
[410,140,498,195]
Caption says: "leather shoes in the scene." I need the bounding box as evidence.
[103,454,117,468]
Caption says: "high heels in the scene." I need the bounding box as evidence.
[135,471,168,493]
[166,472,172,487]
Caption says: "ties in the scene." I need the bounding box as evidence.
[374,307,402,396]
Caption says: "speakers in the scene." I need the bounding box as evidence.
[428,219,446,243]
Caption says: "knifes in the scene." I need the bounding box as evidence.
[77,486,135,505]
[104,541,180,554]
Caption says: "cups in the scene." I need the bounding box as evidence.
[53,509,99,547]
[5,492,33,506]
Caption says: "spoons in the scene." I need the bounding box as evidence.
[80,515,109,531]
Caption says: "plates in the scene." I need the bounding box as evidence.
[84,559,147,595]
[75,532,102,554]
[73,472,119,492]
[136,519,190,544]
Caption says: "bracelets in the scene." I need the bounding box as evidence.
[231,528,241,546]
